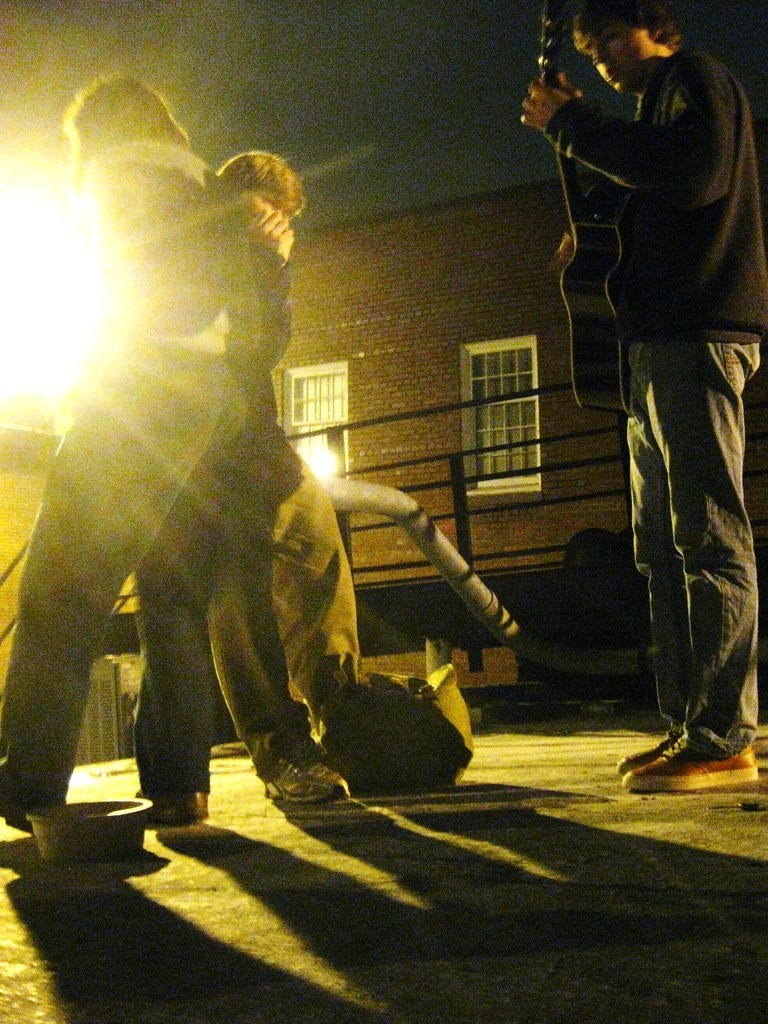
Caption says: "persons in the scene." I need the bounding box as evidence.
[1,74,404,827]
[517,2,768,791]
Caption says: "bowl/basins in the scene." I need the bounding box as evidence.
[27,799,154,864]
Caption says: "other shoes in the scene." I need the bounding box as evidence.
[0,785,66,835]
[134,788,208,824]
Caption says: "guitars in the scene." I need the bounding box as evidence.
[541,1,632,412]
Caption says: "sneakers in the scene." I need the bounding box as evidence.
[617,737,759,793]
[263,758,344,805]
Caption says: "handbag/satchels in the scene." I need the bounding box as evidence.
[324,661,473,796]
